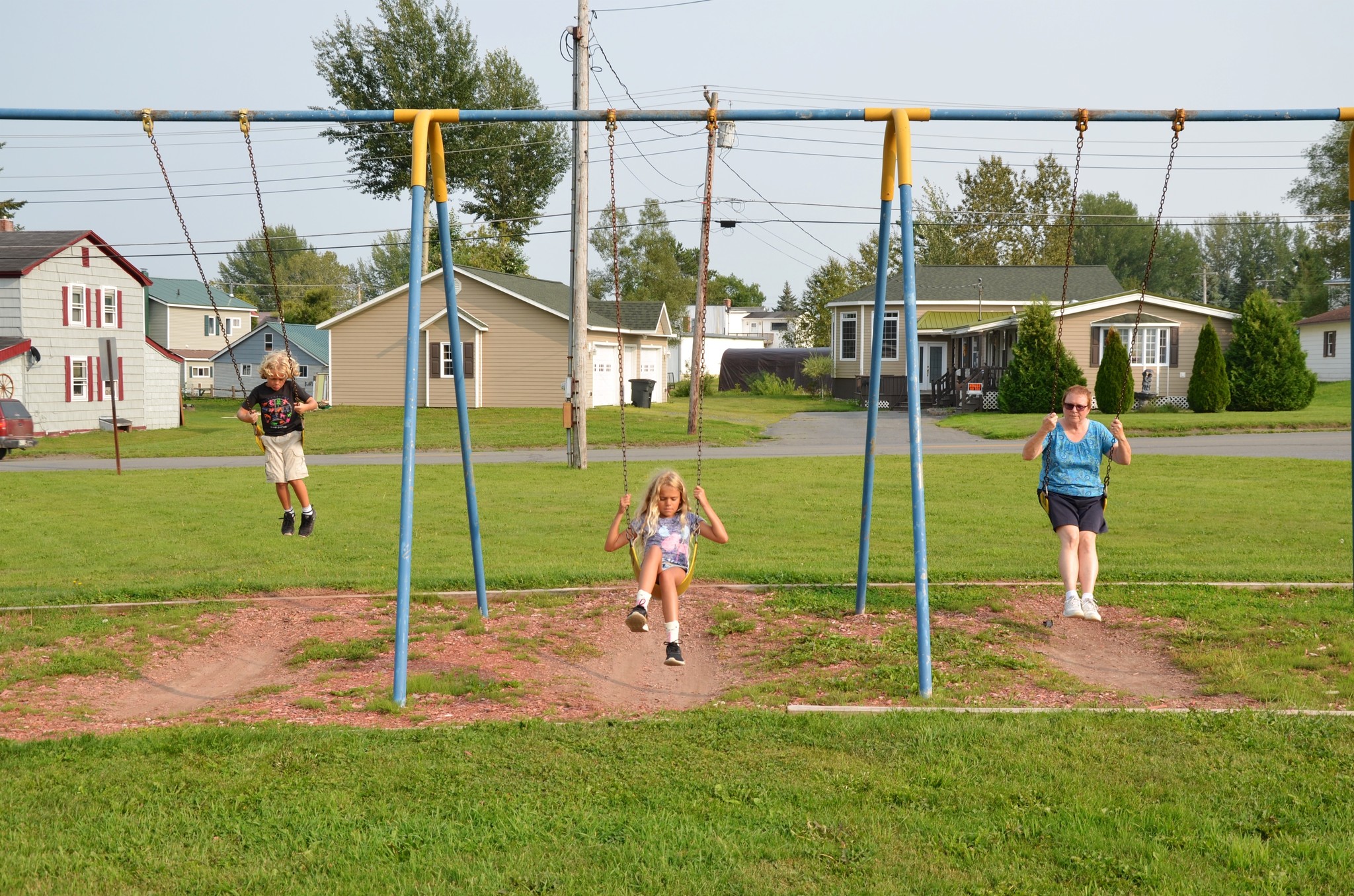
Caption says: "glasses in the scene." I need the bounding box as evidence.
[1064,401,1089,411]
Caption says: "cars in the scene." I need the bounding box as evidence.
[0,398,38,460]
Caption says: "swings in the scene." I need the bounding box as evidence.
[142,118,302,452]
[1037,122,1184,517]
[606,119,719,599]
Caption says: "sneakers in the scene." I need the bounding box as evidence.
[663,639,685,665]
[1062,596,1085,619]
[1081,595,1101,621]
[281,512,296,536]
[298,508,316,537]
[624,599,649,632]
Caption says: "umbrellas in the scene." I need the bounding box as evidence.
[1144,369,1155,374]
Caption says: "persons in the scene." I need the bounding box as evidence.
[1146,373,1153,392]
[1021,385,1132,622]
[605,470,728,666]
[1142,372,1149,392]
[237,352,318,537]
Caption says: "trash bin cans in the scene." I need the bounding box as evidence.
[628,378,657,407]
